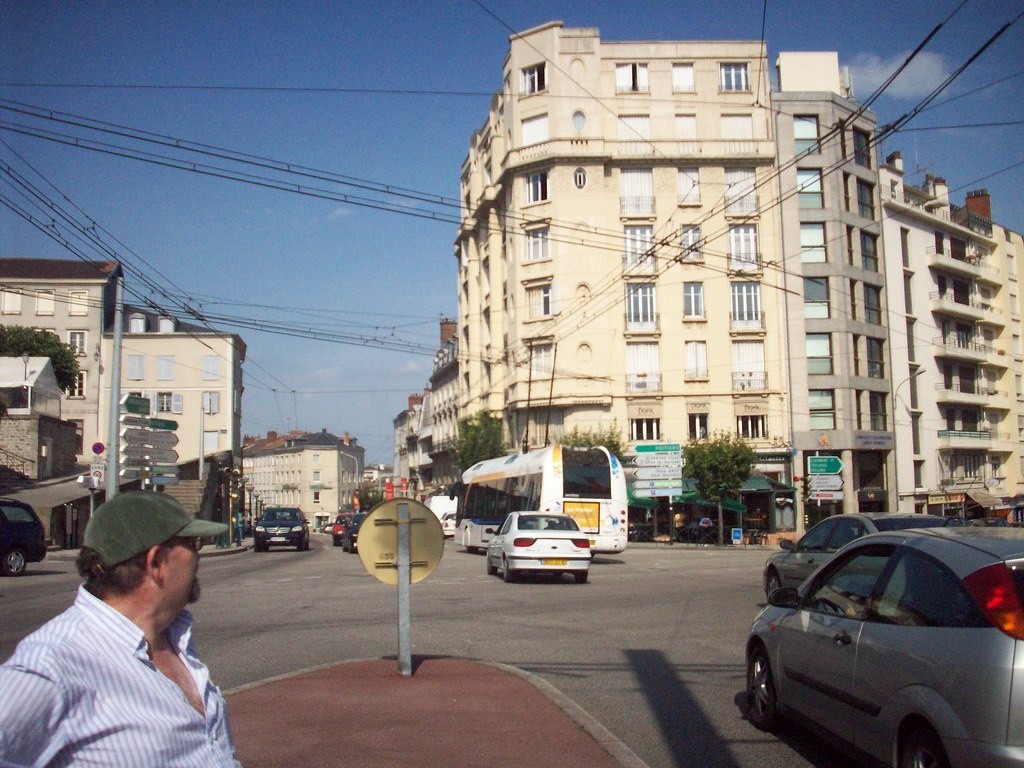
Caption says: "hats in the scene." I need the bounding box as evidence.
[81,489,229,578]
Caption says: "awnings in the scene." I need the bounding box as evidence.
[682,473,774,491]
[965,490,1002,508]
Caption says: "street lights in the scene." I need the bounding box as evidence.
[224,466,240,546]
[262,503,267,513]
[258,499,263,521]
[246,485,255,537]
[891,369,928,510]
[238,476,249,541]
[253,490,261,523]
[340,451,359,513]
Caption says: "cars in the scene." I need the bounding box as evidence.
[743,526,1024,768]
[440,512,456,538]
[485,510,592,583]
[342,514,368,554]
[254,505,311,551]
[320,523,333,533]
[325,525,333,534]
[332,514,352,546]
[761,512,963,595]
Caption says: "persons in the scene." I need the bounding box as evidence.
[0,490,244,768]
[699,518,714,528]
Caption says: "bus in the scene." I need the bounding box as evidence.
[454,444,629,560]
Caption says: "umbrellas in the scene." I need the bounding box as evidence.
[672,492,747,513]
[627,486,660,509]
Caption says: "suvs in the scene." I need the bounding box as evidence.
[0,498,46,577]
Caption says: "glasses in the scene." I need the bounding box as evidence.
[156,535,204,552]
